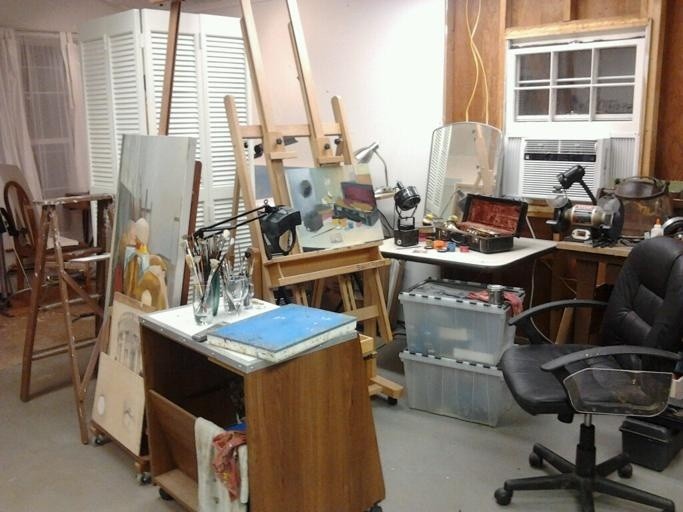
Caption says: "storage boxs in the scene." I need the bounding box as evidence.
[398,277,527,367]
[619,397,683,473]
[399,343,520,428]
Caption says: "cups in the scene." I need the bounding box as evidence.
[488,285,503,308]
[193,272,221,326]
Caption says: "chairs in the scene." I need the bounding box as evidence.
[2,180,89,300]
[494,235,683,511]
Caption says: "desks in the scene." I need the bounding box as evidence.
[377,232,559,288]
[552,234,635,345]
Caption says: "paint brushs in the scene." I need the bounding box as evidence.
[183,233,250,316]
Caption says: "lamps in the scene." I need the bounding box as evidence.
[558,166,598,206]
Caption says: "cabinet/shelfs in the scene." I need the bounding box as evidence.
[137,297,386,512]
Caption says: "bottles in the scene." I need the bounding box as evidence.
[652,218,664,237]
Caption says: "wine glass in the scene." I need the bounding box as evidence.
[226,276,250,320]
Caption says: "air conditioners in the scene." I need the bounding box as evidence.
[516,134,611,204]
